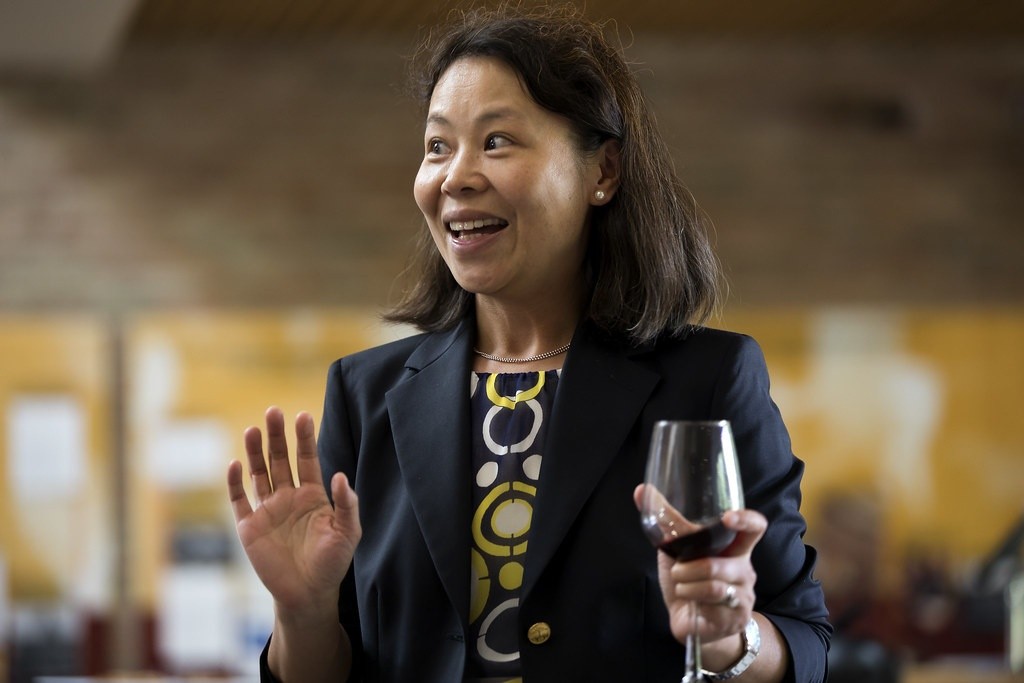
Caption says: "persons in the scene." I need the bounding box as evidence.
[225,0,834,683]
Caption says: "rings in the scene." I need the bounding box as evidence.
[720,585,737,608]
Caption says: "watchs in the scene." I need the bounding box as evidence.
[699,619,761,680]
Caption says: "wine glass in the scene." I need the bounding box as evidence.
[641,419,745,683]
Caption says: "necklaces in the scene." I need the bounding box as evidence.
[474,343,571,363]
